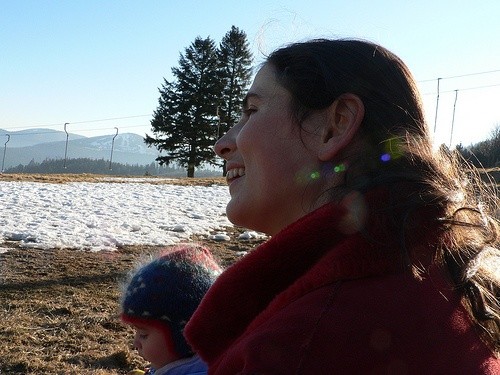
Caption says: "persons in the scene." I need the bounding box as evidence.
[118,245,224,374]
[181,38,500,375]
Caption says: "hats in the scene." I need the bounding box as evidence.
[117,242,224,360]
